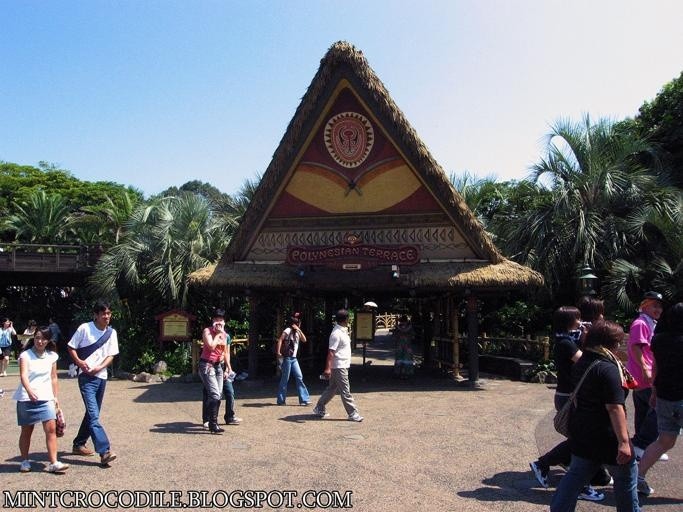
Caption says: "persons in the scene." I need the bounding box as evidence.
[0,315,65,377]
[394,312,416,380]
[202,327,244,430]
[313,310,364,422]
[66,298,122,466]
[529,290,683,512]
[10,325,71,475]
[197,308,229,432]
[275,316,313,406]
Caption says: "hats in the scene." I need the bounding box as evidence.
[643,291,668,303]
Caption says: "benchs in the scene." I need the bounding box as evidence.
[462,345,540,382]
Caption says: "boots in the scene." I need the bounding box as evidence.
[217,400,221,417]
[209,400,224,434]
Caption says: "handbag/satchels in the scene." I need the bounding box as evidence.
[553,400,571,437]
[280,331,295,357]
[56,408,67,437]
[68,348,84,377]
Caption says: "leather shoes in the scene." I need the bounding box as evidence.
[101,452,116,465]
[73,445,95,456]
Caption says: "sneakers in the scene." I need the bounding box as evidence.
[577,486,604,501]
[226,415,243,424]
[313,407,329,416]
[636,477,653,494]
[528,462,551,488]
[300,402,313,406]
[21,460,32,472]
[348,411,364,421]
[658,453,668,461]
[50,462,70,473]
[204,422,210,430]
